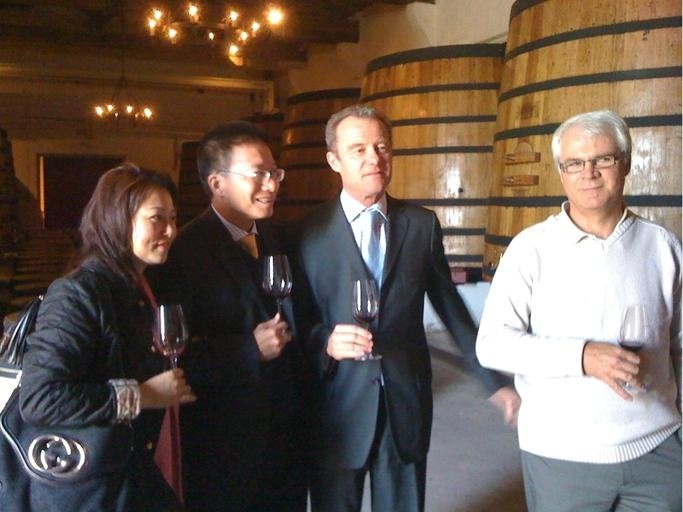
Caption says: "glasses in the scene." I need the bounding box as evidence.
[219,169,285,182]
[559,153,621,174]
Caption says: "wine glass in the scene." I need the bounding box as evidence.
[154,304,188,371]
[261,256,291,321]
[350,279,384,361]
[618,305,652,395]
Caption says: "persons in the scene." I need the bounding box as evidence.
[145,122,312,512]
[0,160,199,511]
[476,111,683,512]
[283,104,522,512]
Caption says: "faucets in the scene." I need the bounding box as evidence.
[4,295,42,367]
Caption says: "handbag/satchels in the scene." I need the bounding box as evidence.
[0,385,131,485]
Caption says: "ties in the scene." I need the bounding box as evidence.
[242,233,260,260]
[360,211,383,292]
